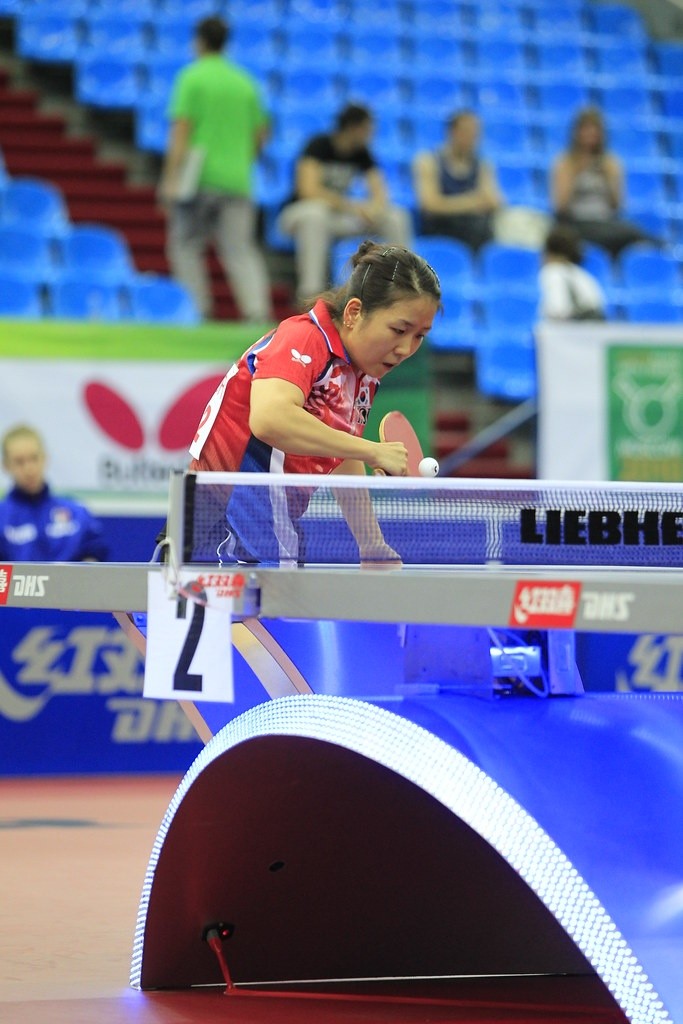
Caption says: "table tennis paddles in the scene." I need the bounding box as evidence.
[372,411,424,477]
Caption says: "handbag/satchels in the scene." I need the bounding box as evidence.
[171,149,205,204]
[529,259,606,324]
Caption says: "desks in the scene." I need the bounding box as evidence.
[0,560,683,1023]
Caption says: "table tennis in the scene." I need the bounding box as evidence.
[418,457,440,477]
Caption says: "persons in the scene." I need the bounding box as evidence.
[153,13,276,326]
[544,105,663,265]
[413,106,504,249]
[0,425,109,562]
[154,237,442,562]
[538,233,607,319]
[274,104,418,312]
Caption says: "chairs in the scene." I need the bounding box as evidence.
[0,0,683,456]
